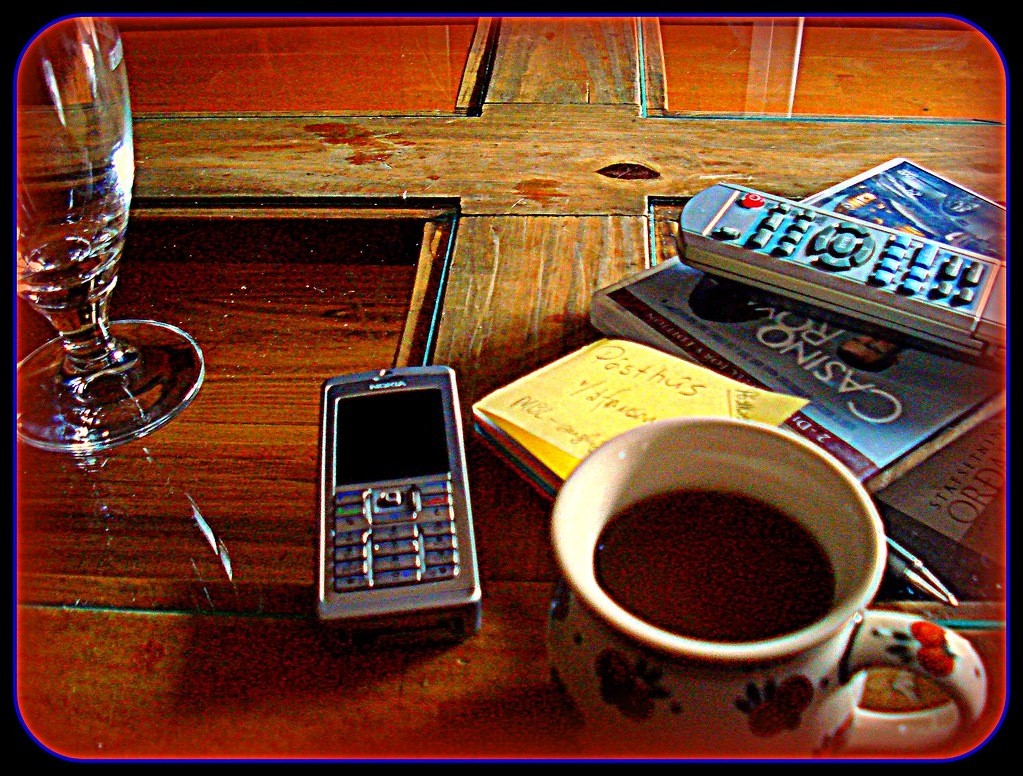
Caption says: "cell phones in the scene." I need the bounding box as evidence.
[315,366,484,639]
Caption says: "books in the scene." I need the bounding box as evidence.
[591,157,1006,495]
[875,412,1007,562]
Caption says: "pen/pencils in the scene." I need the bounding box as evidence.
[886,536,962,608]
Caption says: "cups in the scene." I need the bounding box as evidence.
[543,418,980,752]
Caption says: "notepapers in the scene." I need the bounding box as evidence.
[471,337,814,504]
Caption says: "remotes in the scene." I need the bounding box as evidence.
[678,182,1007,369]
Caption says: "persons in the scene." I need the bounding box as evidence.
[835,334,903,373]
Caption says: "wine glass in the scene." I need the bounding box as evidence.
[18,19,202,455]
